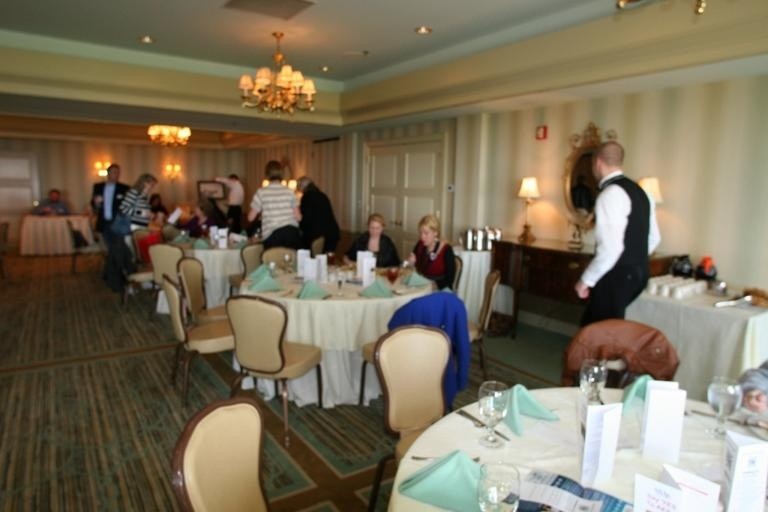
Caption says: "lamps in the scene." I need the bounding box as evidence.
[519,176,540,243]
[241,33,318,119]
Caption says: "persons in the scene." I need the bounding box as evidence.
[186,160,339,258]
[343,214,400,268]
[29,189,71,216]
[92,167,170,292]
[408,214,455,291]
[737,358,768,412]
[573,140,664,329]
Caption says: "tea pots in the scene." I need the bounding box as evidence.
[455,224,501,251]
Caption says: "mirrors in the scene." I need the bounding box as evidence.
[563,124,617,229]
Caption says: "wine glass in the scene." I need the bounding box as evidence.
[476,380,510,451]
[475,461,522,512]
[702,371,742,441]
[266,250,412,289]
[575,358,609,400]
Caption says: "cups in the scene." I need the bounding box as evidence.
[199,222,249,249]
[645,270,706,302]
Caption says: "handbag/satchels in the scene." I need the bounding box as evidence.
[111,213,130,235]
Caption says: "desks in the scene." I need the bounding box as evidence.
[624,274,766,401]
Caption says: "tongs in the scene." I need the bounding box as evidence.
[715,293,753,309]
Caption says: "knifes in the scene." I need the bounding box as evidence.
[455,410,511,444]
